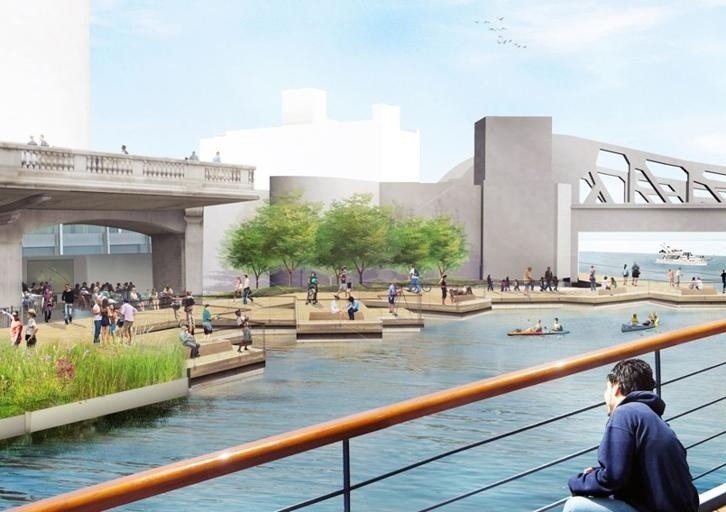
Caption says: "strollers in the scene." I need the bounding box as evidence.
[305,283,318,305]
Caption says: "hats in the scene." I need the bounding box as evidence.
[235,309,241,315]
[26,308,36,316]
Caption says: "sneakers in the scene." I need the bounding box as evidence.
[191,353,200,358]
[237,347,249,352]
[65,321,73,325]
[92,338,100,343]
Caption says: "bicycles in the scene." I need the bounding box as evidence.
[407,278,433,292]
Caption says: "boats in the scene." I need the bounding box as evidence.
[508,328,570,337]
[654,242,709,267]
[621,320,657,331]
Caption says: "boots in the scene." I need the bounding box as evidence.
[44,310,51,322]
